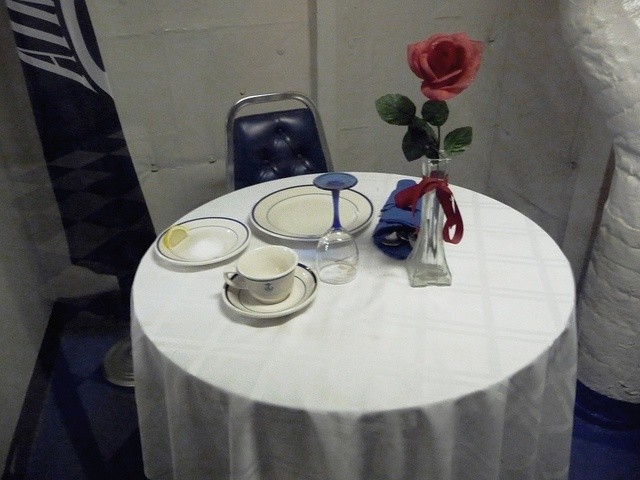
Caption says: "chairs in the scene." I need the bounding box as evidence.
[222,90,336,196]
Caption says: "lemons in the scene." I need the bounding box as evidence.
[163,227,188,250]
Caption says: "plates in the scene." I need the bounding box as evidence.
[155,216,249,268]
[249,182,374,244]
[221,263,318,319]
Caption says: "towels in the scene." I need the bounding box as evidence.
[373,179,421,260]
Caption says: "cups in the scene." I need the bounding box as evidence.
[223,245,300,304]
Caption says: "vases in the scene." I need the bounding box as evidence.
[407,151,453,286]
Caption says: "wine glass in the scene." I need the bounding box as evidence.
[313,173,359,286]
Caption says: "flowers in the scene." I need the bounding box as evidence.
[377,31,486,262]
